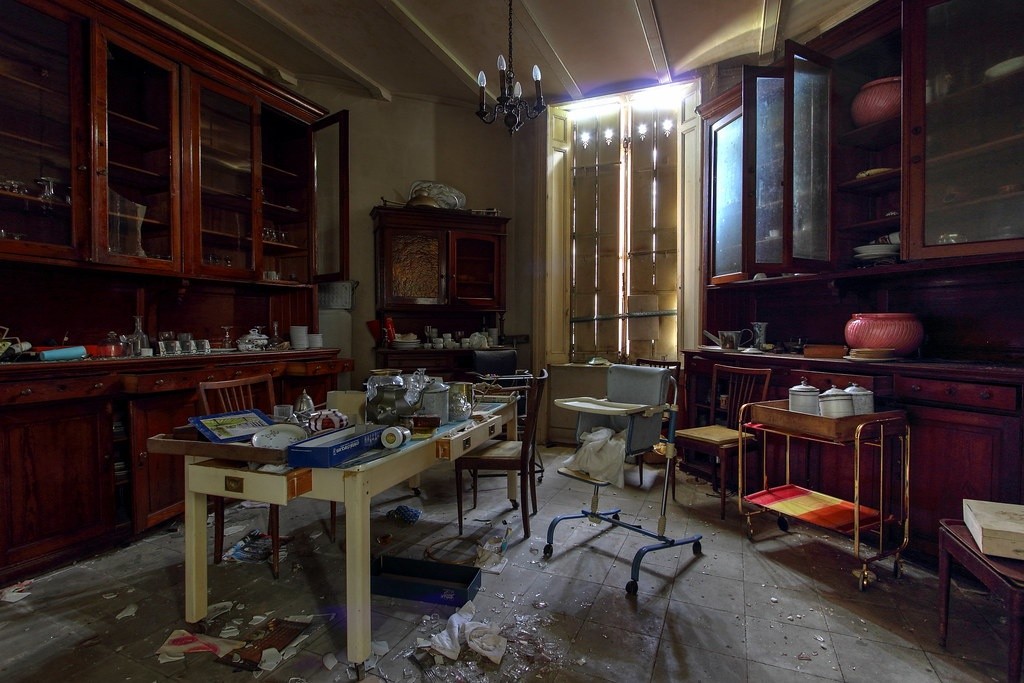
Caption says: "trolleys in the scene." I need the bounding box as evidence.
[737,396,912,593]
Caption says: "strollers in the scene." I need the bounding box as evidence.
[542,363,702,595]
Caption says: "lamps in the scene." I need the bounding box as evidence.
[477,2,552,139]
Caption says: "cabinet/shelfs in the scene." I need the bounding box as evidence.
[828,1,1024,281]
[4,347,339,586]
[370,201,508,316]
[692,32,838,285]
[0,0,184,276]
[186,39,357,290]
[379,347,512,378]
[688,339,1023,570]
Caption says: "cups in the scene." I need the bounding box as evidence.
[787,377,874,419]
[489,328,498,345]
[420,380,450,425]
[424,344,431,348]
[262,227,290,244]
[718,328,753,351]
[481,327,494,347]
[158,330,210,356]
[272,404,294,424]
[462,338,471,348]
[432,333,460,350]
[140,348,152,356]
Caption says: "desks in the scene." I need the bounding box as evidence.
[165,390,525,678]
[935,516,1023,682]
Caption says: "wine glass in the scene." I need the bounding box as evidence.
[1,168,62,203]
[424,326,432,343]
[129,313,149,356]
[219,326,234,348]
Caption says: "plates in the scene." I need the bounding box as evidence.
[252,422,307,449]
[842,355,902,363]
[212,348,237,353]
[114,461,130,476]
[983,55,1024,77]
[852,245,900,260]
[698,346,745,352]
[392,339,421,348]
[290,324,324,351]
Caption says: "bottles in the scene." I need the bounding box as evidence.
[0,340,33,364]
[269,321,285,350]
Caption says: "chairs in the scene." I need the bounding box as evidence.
[200,366,340,571]
[672,361,774,521]
[455,364,550,538]
